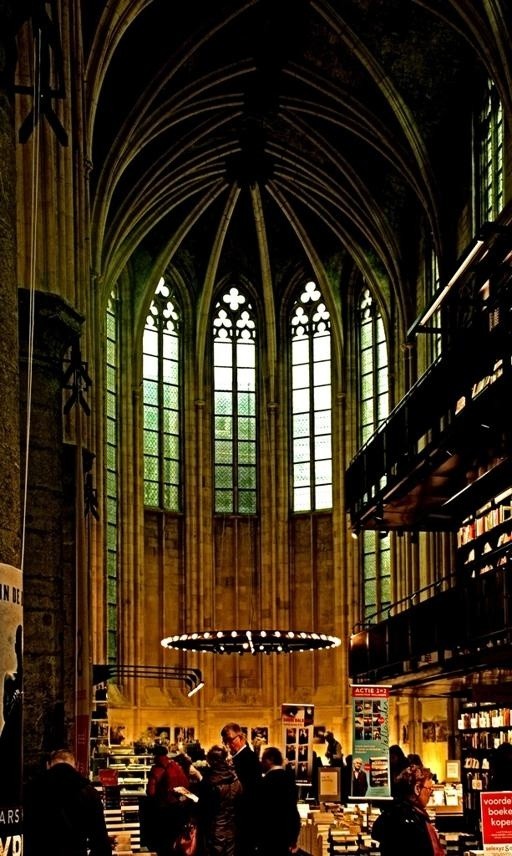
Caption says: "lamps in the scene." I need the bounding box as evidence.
[93,665,211,699]
[158,629,346,656]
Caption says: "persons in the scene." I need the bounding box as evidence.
[133,719,422,856]
[2,625,23,723]
[370,763,444,856]
[24,749,113,856]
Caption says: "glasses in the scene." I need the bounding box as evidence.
[221,734,238,745]
[418,784,434,795]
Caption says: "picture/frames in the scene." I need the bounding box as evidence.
[317,767,342,803]
[456,693,510,839]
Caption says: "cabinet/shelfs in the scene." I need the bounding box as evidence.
[441,464,510,630]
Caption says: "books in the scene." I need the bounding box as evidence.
[94,742,152,856]
[456,499,510,577]
[431,702,512,812]
